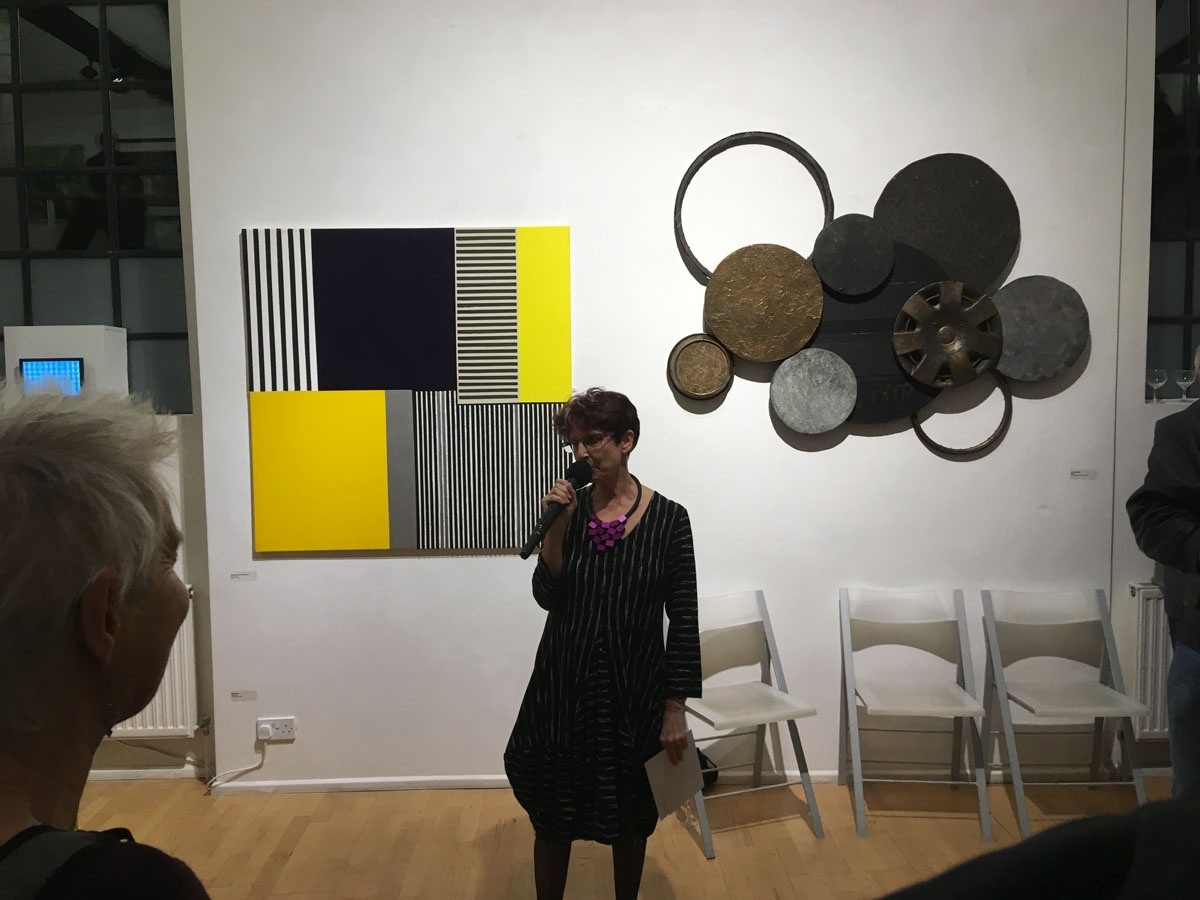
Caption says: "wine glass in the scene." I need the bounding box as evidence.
[1173,370,1196,404]
[1145,369,1168,404]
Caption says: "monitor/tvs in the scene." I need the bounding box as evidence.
[19,357,84,399]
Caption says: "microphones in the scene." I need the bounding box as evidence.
[519,461,592,560]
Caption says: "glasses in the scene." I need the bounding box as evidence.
[561,431,614,453]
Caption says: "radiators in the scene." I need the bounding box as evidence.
[1131,585,1176,741]
[83,585,207,777]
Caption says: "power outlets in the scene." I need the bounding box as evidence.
[255,717,297,741]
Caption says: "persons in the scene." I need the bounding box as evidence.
[1125,369,1200,802]
[2,384,212,900]
[503,389,704,900]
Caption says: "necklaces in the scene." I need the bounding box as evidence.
[587,473,642,552]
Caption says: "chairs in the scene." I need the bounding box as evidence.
[981,586,1148,842]
[840,585,997,844]
[663,588,825,862]
